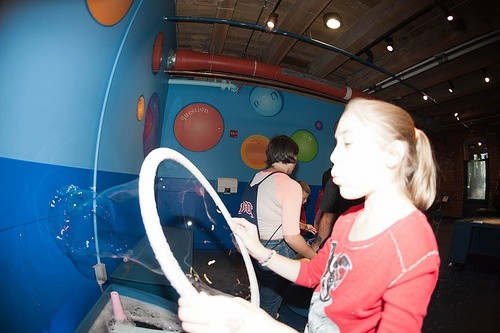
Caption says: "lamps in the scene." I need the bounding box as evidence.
[442,9,453,21]
[448,80,454,93]
[323,12,344,31]
[267,13,278,29]
[385,36,394,52]
[421,89,428,101]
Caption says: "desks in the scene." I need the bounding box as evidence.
[109,227,194,302]
[447,216,500,268]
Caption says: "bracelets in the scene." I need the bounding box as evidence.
[258,249,277,266]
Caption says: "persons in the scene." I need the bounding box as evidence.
[177,97,440,333]
[296,180,317,238]
[309,166,365,257]
[238,134,322,320]
